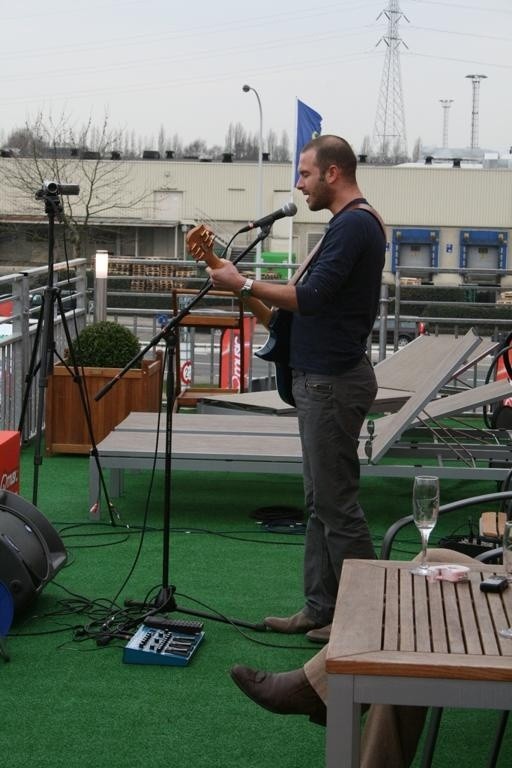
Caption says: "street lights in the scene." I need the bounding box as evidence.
[240,84,266,279]
[465,74,489,149]
[440,98,454,148]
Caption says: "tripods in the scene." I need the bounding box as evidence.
[12,249,116,533]
[83,239,272,648]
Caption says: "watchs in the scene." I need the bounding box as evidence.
[241,278,255,297]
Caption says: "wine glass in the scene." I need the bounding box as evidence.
[410,475,443,576]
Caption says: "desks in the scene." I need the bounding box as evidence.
[319,556,511,766]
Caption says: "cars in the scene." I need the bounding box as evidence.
[164,306,258,339]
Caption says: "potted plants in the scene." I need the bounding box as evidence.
[45,317,164,456]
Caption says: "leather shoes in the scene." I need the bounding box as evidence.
[306,620,331,643]
[229,663,327,728]
[263,611,321,635]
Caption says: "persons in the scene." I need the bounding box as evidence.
[204,134,386,641]
[228,546,512,768]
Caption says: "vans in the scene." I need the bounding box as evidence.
[371,315,430,347]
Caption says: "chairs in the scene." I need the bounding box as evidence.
[87,328,512,522]
[373,488,511,765]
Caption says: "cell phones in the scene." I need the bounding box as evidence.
[479,574,508,592]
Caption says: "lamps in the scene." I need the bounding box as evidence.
[93,247,112,280]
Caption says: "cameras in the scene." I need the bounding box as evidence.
[35,180,80,197]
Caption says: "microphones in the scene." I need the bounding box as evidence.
[238,202,298,238]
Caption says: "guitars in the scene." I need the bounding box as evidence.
[187,226,294,405]
